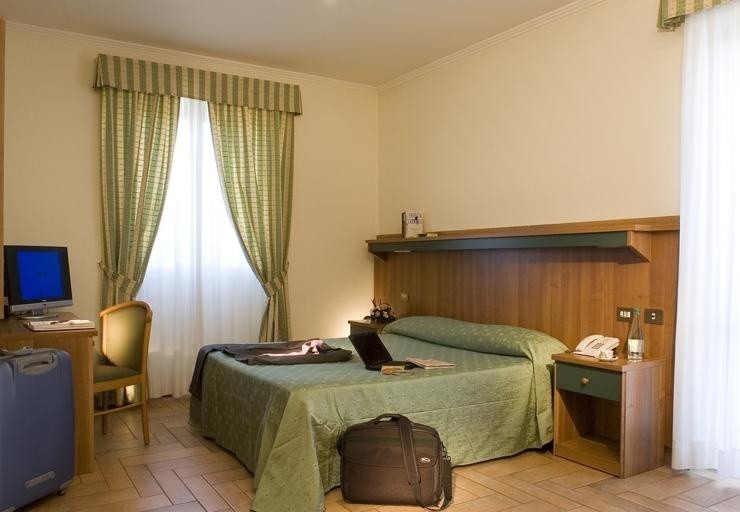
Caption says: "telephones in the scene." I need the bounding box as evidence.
[572,334,620,358]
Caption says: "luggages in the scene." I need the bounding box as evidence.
[0,348,75,512]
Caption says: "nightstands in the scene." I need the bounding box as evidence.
[348,319,384,336]
[551,352,668,478]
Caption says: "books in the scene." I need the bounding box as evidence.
[407,357,456,370]
[402,212,423,238]
[381,365,405,375]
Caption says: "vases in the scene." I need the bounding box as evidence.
[376,316,387,324]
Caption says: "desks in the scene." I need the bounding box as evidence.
[0,312,98,476]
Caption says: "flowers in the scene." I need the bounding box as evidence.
[363,297,400,324]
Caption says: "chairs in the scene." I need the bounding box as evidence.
[92,301,153,445]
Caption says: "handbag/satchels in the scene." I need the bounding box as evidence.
[340,422,442,506]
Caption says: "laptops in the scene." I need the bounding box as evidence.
[348,331,418,370]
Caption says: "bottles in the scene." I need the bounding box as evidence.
[625,306,643,361]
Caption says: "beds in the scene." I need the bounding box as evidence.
[188,216,680,512]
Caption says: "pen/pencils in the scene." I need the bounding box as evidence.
[51,319,69,324]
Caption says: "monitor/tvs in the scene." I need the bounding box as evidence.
[3,245,73,320]
